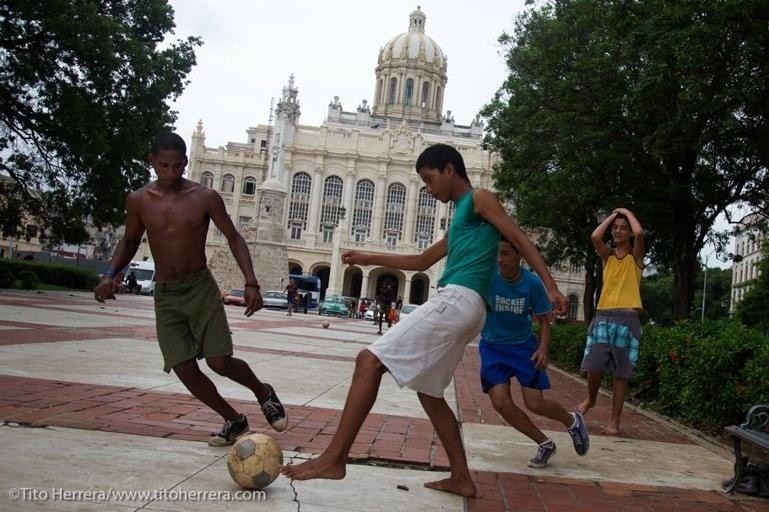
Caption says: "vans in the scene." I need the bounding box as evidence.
[122,262,157,295]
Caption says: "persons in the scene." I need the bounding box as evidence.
[480,230,589,466]
[276,142,571,498]
[575,208,645,434]
[94,130,288,445]
[347,282,402,335]
[282,278,297,316]
[125,272,142,295]
[292,294,301,313]
[114,272,125,295]
[302,291,313,314]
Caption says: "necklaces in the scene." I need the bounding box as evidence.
[497,264,522,281]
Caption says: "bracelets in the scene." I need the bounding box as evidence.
[102,270,117,277]
[244,283,261,289]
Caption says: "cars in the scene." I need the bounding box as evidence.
[317,295,421,322]
[262,290,289,308]
[224,287,249,306]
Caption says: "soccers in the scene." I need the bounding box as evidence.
[227,434,282,488]
[323,321,328,327]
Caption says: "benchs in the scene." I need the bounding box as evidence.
[721,403,769,511]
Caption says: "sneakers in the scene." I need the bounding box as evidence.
[207,413,249,447]
[527,438,556,467]
[566,412,589,457]
[257,383,287,432]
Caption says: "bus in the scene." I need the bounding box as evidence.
[290,274,321,308]
